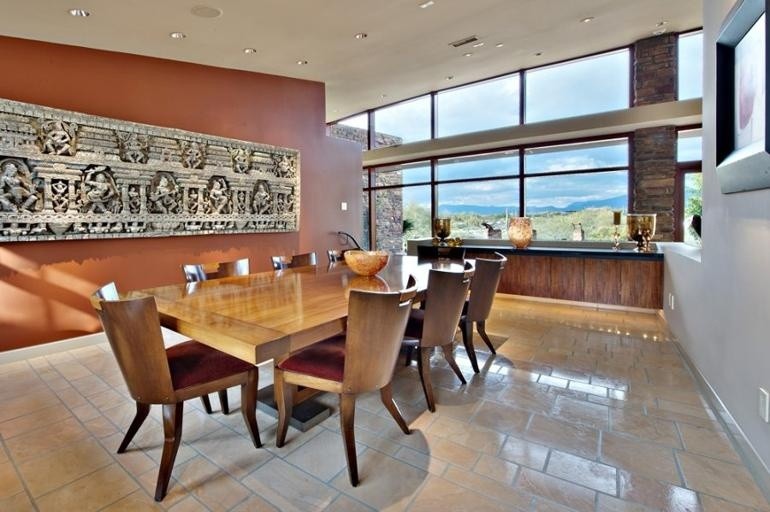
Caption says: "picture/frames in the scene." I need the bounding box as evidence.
[713,0,770,197]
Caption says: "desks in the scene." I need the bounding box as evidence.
[121,254,477,458]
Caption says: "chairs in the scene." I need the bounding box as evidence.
[326,248,361,262]
[182,258,251,281]
[415,244,467,262]
[421,251,506,375]
[403,261,476,413]
[89,278,264,504]
[271,252,316,271]
[269,275,419,488]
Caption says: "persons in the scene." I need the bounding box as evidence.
[288,194,294,213]
[85,168,114,214]
[233,148,251,173]
[276,194,283,212]
[41,117,71,156]
[251,181,271,213]
[237,190,244,212]
[51,182,67,210]
[187,188,197,212]
[208,178,229,212]
[2,164,37,212]
[123,130,147,163]
[183,138,202,167]
[125,187,140,212]
[147,176,178,210]
[277,154,294,176]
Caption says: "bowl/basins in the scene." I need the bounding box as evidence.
[343,249,391,276]
[342,274,392,300]
